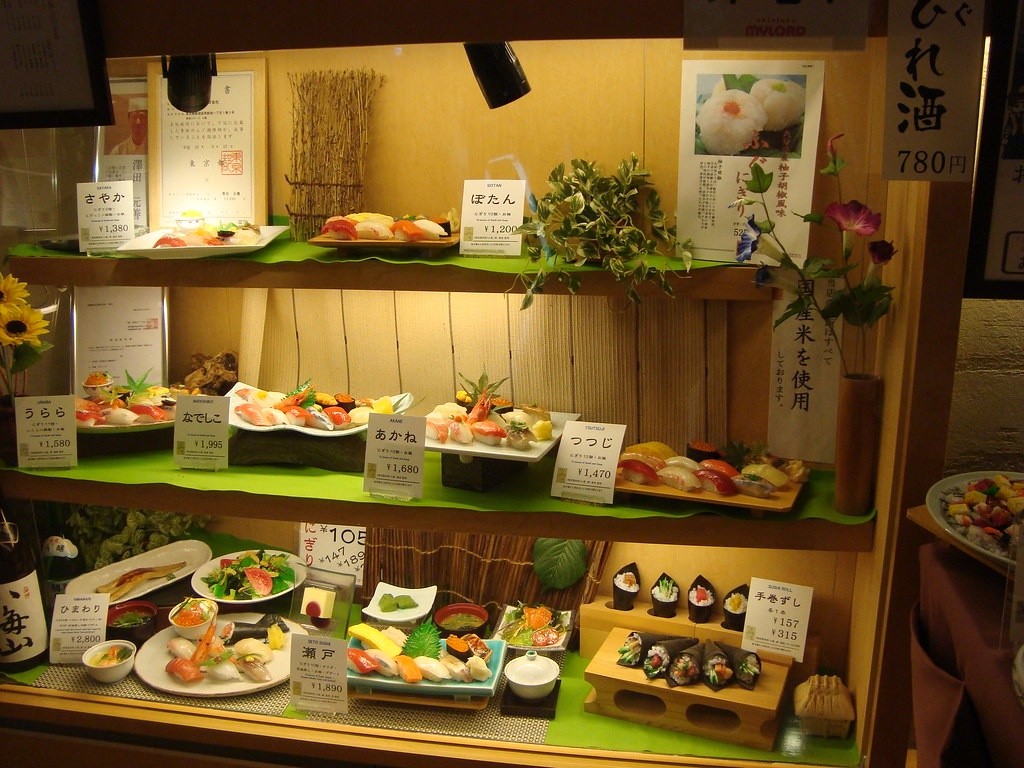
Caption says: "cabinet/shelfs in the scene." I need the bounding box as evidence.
[0,0,991,768]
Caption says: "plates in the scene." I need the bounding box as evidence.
[926,472,1024,567]
[362,582,437,625]
[419,402,581,460]
[613,454,810,517]
[493,606,572,651]
[307,226,460,259]
[192,549,307,606]
[348,633,507,701]
[224,381,413,436]
[65,540,211,606]
[134,613,310,698]
[75,393,176,434]
[117,225,291,259]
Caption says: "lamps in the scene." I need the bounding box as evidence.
[161,53,216,113]
[463,38,532,108]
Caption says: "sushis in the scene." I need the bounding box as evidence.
[153,225,261,248]
[76,385,200,426]
[346,623,493,682]
[612,562,762,690]
[322,212,453,242]
[235,388,395,431]
[617,440,789,498]
[165,612,287,682]
[426,391,553,449]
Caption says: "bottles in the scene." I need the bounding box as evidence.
[41,500,87,622]
[1,481,50,675]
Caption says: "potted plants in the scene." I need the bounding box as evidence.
[509,150,694,310]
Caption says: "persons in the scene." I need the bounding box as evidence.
[110,98,148,155]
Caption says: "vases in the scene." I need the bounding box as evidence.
[0,394,30,465]
[835,371,880,516]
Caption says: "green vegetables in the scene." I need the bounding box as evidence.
[117,647,132,662]
[199,548,296,601]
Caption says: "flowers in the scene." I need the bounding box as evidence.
[728,132,896,378]
[0,270,55,406]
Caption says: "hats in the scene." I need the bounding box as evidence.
[128,97,150,114]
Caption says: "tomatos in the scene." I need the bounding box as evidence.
[243,568,273,596]
[220,558,233,568]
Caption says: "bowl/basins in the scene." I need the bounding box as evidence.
[81,640,137,684]
[504,649,560,701]
[168,598,218,640]
[109,602,159,644]
[82,379,113,397]
[434,603,489,638]
